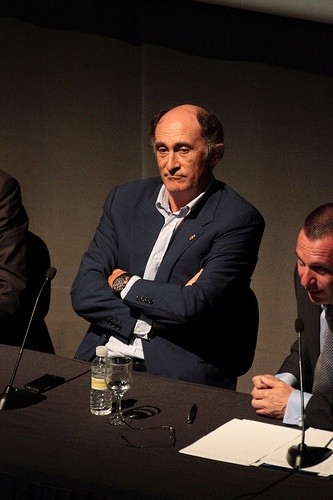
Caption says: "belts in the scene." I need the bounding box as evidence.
[113,357,147,372]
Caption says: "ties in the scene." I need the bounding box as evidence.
[311,307,333,394]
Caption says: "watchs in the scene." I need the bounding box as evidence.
[113,273,133,293]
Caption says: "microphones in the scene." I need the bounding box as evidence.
[285,319,332,469]
[1,267,58,410]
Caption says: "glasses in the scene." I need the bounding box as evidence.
[121,420,176,449]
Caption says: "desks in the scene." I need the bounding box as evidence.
[0,345,333,500]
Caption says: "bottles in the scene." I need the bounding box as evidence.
[90,346,114,416]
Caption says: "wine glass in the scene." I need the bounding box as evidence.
[104,355,134,426]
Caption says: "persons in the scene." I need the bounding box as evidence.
[0,169,56,354]
[251,203,333,432]
[70,105,266,392]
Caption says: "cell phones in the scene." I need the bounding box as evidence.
[24,374,65,394]
